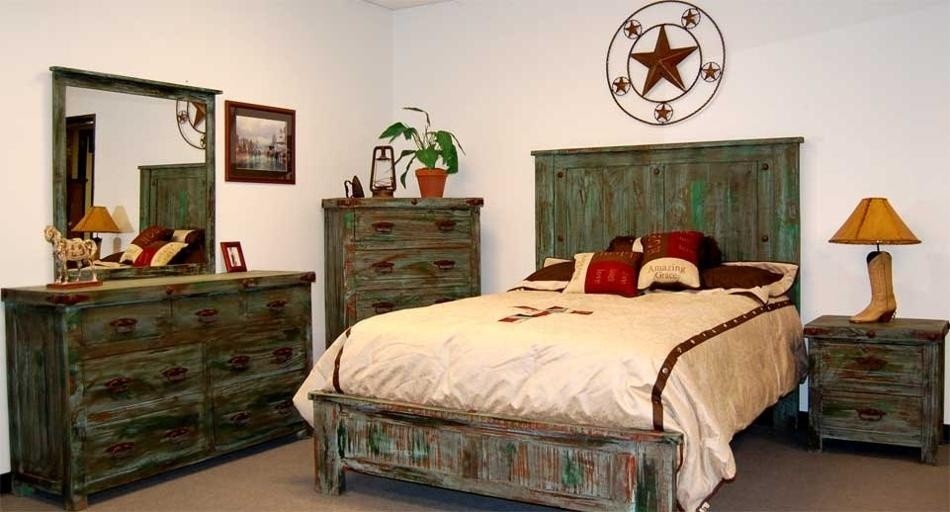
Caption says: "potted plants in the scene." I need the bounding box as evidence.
[378,107,465,197]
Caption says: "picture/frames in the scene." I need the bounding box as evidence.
[222,241,248,273]
[225,101,296,185]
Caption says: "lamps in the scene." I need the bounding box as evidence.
[828,197,922,323]
[370,146,397,196]
[72,206,118,264]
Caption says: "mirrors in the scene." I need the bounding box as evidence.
[49,66,223,283]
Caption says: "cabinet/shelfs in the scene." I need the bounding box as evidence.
[323,197,482,347]
[0,269,317,511]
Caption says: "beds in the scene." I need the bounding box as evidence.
[295,137,805,512]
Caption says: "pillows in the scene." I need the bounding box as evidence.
[508,231,799,304]
[118,224,203,268]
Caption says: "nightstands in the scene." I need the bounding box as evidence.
[802,316,950,464]
